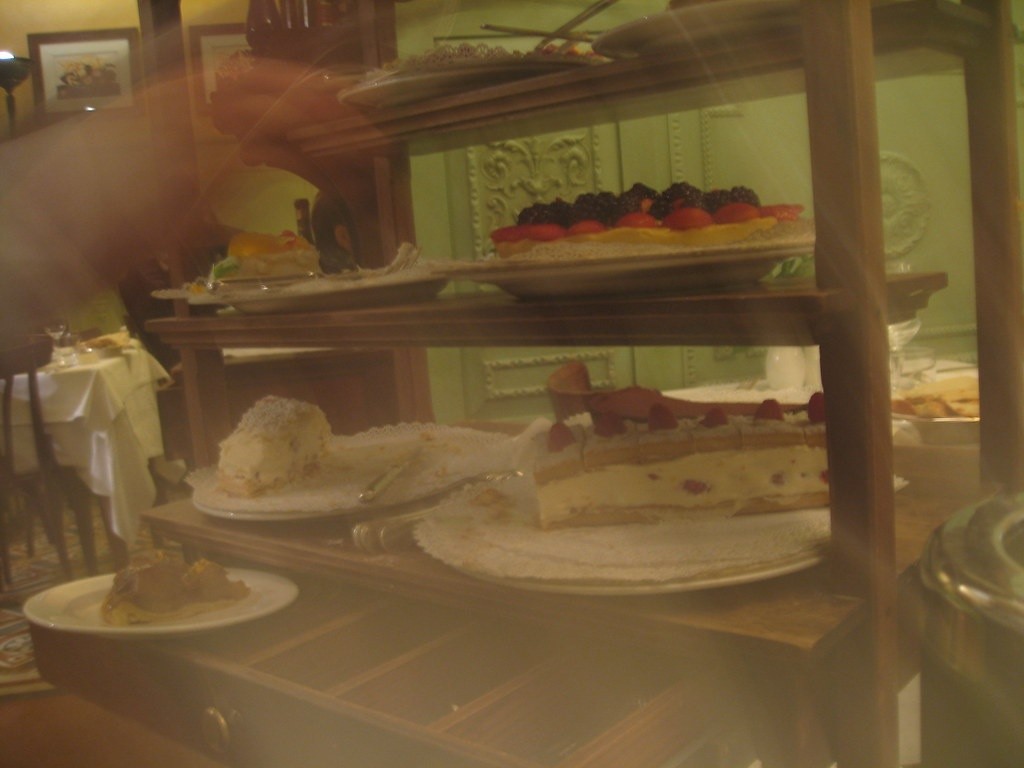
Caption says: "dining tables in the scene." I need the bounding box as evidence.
[1,340,173,575]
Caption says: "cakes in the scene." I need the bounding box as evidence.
[888,374,980,418]
[486,179,805,258]
[214,395,337,498]
[528,361,830,527]
[100,547,253,628]
[209,230,321,285]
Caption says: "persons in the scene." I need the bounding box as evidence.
[0,58,362,349]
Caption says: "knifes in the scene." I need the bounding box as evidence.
[363,438,429,502]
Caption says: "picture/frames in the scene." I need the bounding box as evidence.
[188,23,256,118]
[26,26,143,123]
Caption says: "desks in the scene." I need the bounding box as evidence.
[661,356,981,413]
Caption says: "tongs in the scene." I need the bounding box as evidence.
[351,469,523,553]
[207,271,335,302]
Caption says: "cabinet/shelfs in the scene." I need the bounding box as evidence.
[23,0,1024,768]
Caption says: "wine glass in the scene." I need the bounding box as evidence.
[883,260,936,396]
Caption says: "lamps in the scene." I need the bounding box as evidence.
[0,51,33,134]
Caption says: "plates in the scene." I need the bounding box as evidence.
[592,0,830,63]
[198,250,457,310]
[336,44,603,117]
[451,213,814,297]
[22,562,300,640]
[413,444,909,598]
[188,422,514,522]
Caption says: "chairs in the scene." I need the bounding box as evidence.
[0,333,95,592]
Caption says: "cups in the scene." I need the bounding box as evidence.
[43,322,80,368]
